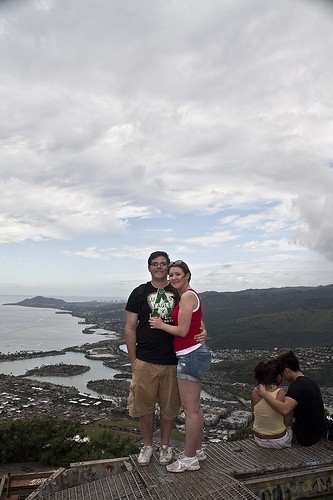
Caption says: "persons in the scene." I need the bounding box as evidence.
[125,251,211,472]
[251,351,324,450]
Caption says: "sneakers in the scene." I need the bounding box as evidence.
[137,446,153,465]
[173,449,206,461]
[165,454,200,472]
[158,444,173,465]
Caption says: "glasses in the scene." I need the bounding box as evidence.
[168,260,182,266]
[149,262,168,267]
